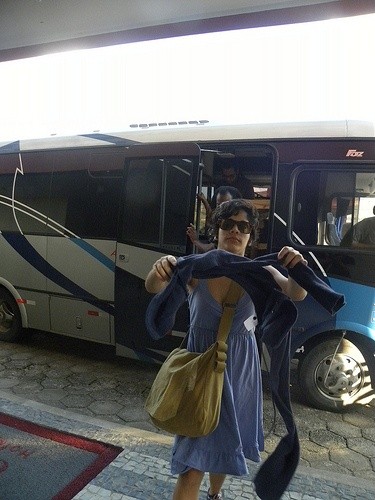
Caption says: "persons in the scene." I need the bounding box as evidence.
[340,205,375,250]
[208,158,255,198]
[144,199,308,500]
[187,186,243,253]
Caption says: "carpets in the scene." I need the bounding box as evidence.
[0,411,124,500]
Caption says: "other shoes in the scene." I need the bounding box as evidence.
[207,488,223,500]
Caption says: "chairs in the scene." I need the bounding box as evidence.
[326,212,341,245]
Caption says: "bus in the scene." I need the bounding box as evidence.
[1,118,375,412]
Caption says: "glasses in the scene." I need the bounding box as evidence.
[218,218,254,234]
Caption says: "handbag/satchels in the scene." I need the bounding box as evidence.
[144,341,228,437]
